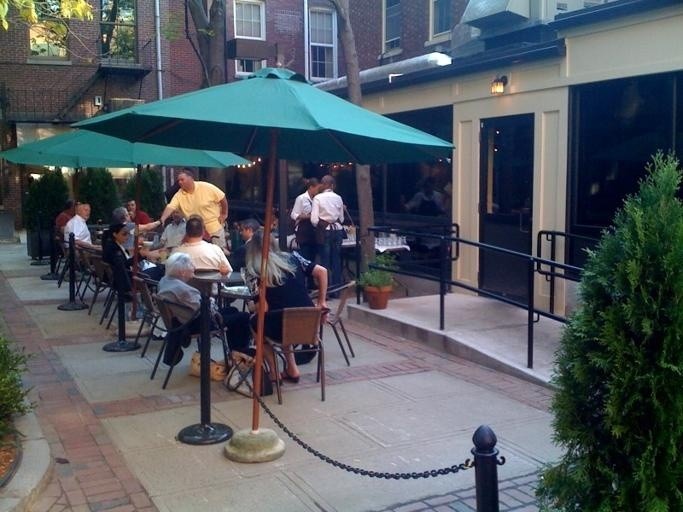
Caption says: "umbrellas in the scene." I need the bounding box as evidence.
[70,63,455,429]
[0,130,253,320]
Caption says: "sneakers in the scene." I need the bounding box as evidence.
[326,290,341,299]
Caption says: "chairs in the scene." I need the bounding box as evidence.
[43,219,375,391]
[260,307,331,404]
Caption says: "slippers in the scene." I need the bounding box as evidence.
[267,373,282,385]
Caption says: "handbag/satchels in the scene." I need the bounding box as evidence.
[222,357,274,399]
[150,312,168,340]
[188,350,226,380]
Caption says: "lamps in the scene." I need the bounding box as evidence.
[489,71,508,97]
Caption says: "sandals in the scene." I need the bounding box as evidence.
[280,370,299,383]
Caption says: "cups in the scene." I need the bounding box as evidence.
[142,241,152,248]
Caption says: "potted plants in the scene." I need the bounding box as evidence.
[20,165,71,260]
[360,254,397,310]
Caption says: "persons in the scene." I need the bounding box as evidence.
[388,175,452,219]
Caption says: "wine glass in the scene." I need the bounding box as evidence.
[240,268,247,292]
